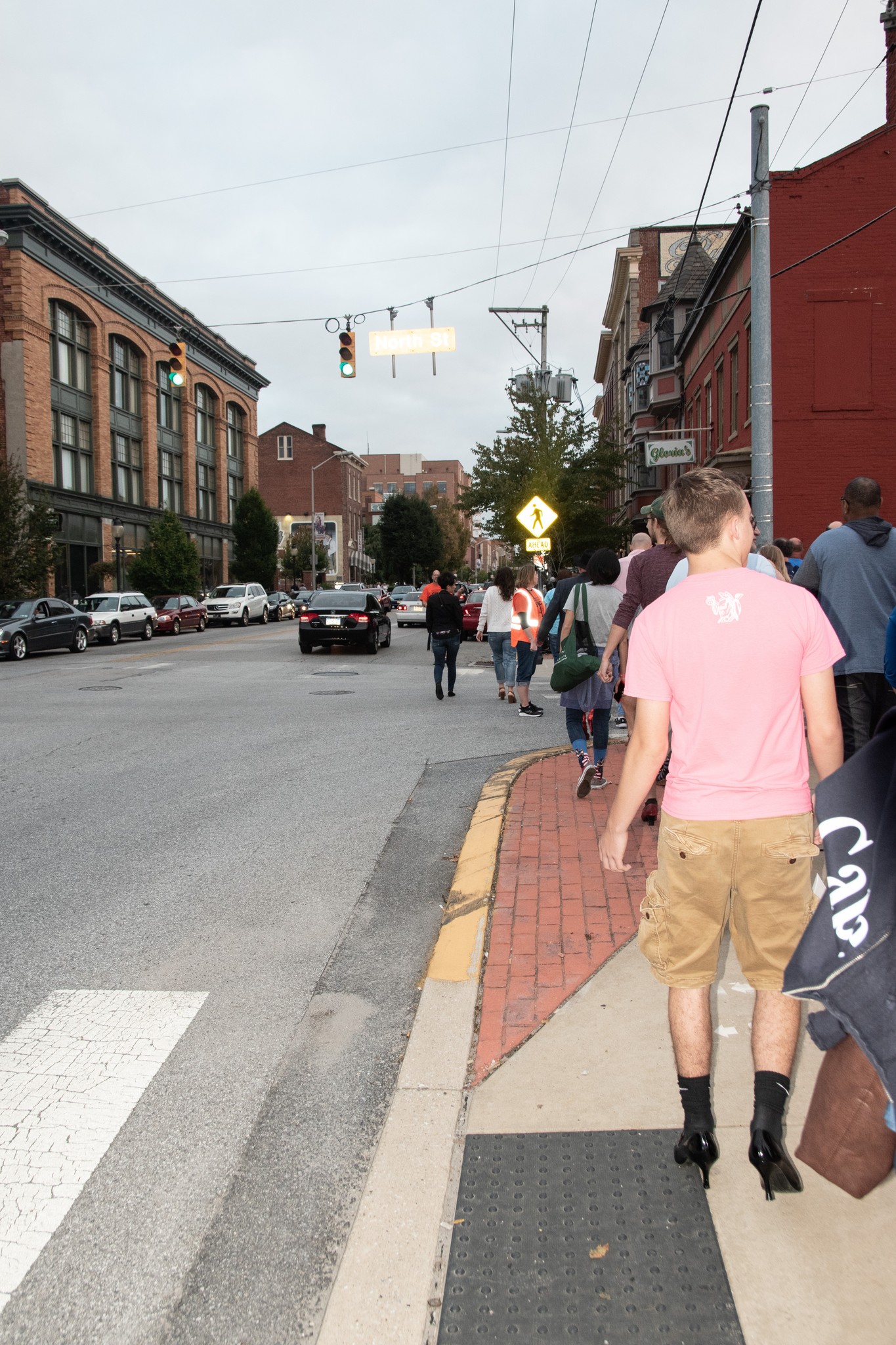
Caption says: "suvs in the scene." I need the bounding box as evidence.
[339,582,369,592]
[200,581,270,627]
[75,591,158,646]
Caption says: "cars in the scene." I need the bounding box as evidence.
[389,585,417,609]
[0,597,91,661]
[287,590,318,618]
[462,590,488,641]
[419,583,428,591]
[299,590,393,655]
[453,581,485,607]
[149,595,210,636]
[266,590,296,622]
[396,591,426,628]
[299,592,317,616]
[358,588,392,615]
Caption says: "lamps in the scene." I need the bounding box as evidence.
[304,512,309,515]
[286,512,291,515]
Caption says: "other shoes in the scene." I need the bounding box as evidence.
[436,683,444,700]
[447,691,455,697]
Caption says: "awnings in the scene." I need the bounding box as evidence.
[348,551,375,573]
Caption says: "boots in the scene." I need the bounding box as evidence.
[587,708,594,736]
[582,712,589,740]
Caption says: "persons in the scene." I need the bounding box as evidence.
[376,582,388,592]
[599,465,848,1202]
[419,478,896,825]
[280,582,323,591]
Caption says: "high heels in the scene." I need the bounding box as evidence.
[674,1133,720,1189]
[641,804,658,825]
[656,771,668,785]
[507,691,517,704]
[498,688,506,700]
[748,1129,804,1202]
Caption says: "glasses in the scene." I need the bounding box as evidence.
[643,517,660,524]
[841,497,849,505]
[750,521,757,530]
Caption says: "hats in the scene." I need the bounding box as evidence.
[640,495,667,520]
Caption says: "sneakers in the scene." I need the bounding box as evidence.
[519,706,543,717]
[590,777,607,789]
[518,701,543,712]
[615,717,627,728]
[576,761,596,798]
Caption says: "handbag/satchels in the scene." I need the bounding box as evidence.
[550,585,601,692]
[795,1032,896,1199]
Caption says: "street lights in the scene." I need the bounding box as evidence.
[289,543,298,586]
[311,451,354,590]
[111,517,125,592]
[500,551,507,568]
[491,547,503,582]
[475,539,490,584]
[370,557,376,588]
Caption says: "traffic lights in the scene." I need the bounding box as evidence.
[168,341,186,388]
[339,332,358,382]
